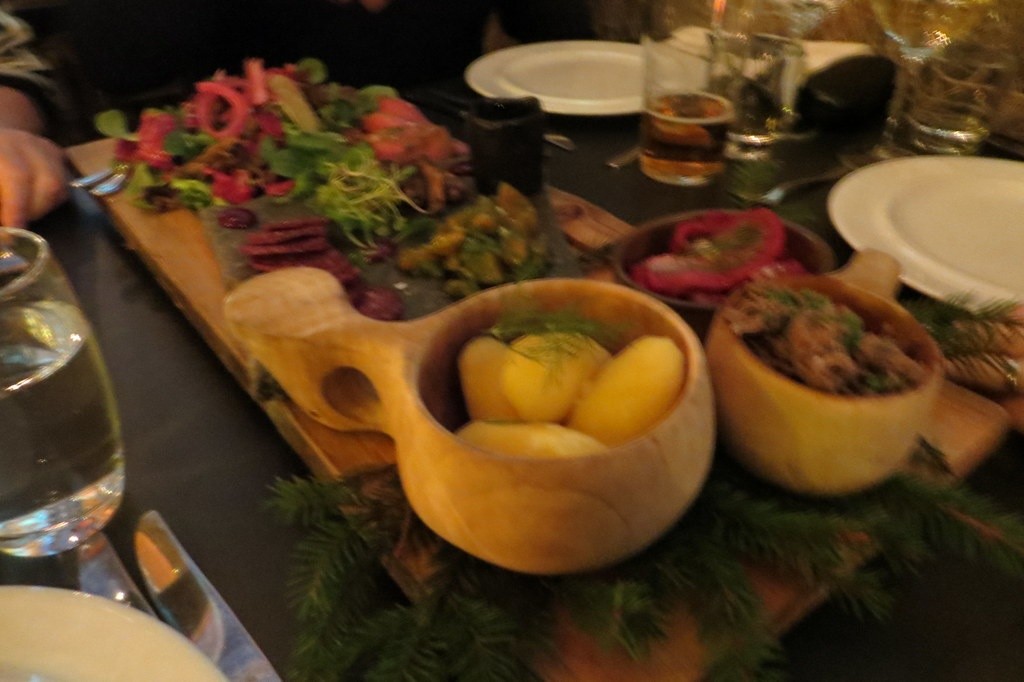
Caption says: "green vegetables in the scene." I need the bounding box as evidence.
[93,58,431,252]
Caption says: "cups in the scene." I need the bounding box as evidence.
[640,33,748,185]
[713,34,805,159]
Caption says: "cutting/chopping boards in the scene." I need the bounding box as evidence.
[67,89,630,488]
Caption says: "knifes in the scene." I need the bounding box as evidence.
[135,509,279,682]
[76,532,157,620]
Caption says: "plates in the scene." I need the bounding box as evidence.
[0,585,229,682]
[828,155,1024,313]
[467,41,709,115]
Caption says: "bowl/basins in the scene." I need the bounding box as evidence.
[615,214,834,330]
[708,250,939,493]
[223,267,712,575]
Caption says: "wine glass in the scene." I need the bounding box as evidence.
[0,227,152,617]
[844,0,1024,170]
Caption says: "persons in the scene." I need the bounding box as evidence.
[0,0,68,244]
[57,0,595,93]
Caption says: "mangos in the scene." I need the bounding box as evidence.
[448,327,687,456]
